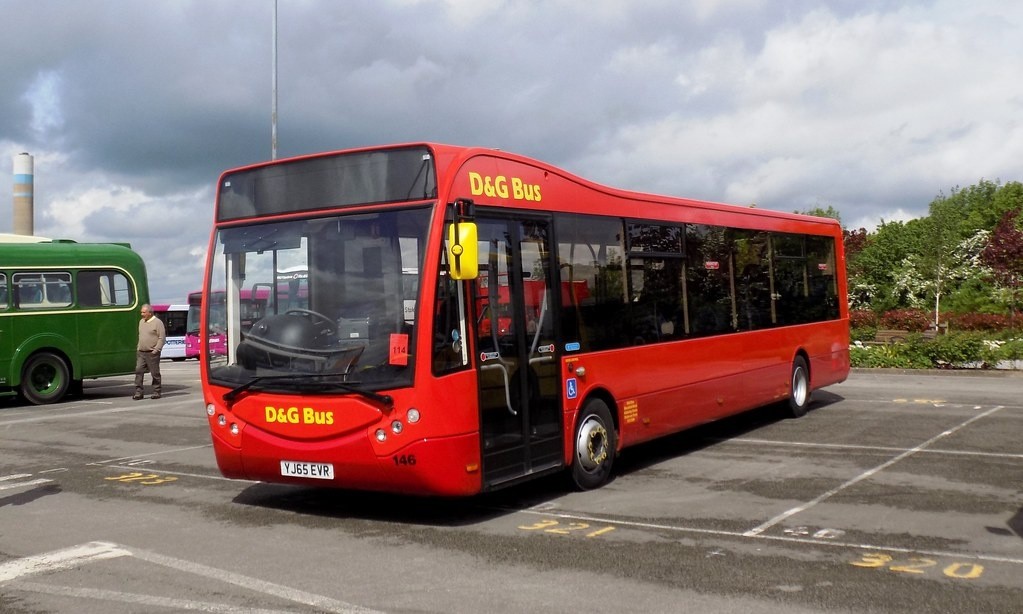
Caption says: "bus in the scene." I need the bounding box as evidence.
[250,265,456,327]
[150,303,190,361]
[185,284,310,361]
[0,238,150,406]
[197,140,852,501]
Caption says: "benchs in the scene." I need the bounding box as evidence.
[863,329,938,347]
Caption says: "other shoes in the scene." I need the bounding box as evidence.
[151,393,160,399]
[132,394,143,400]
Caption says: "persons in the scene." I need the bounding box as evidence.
[132,304,166,399]
